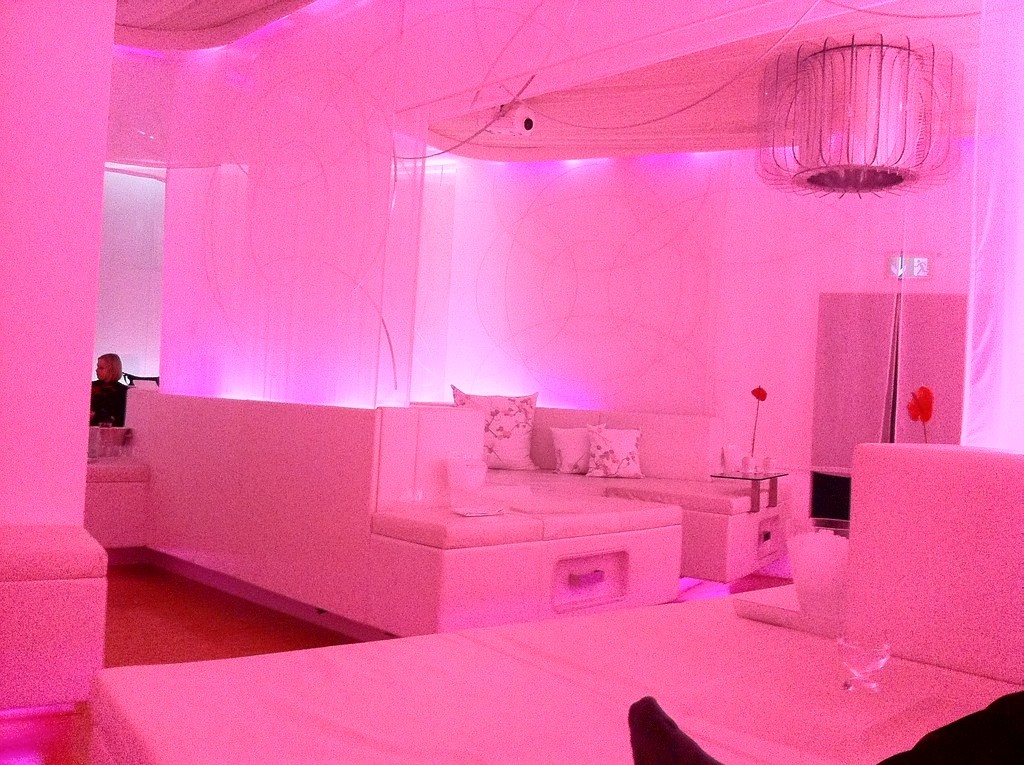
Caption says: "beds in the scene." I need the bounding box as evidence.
[89,440,1024,765]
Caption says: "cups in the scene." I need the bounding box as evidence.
[786,516,850,616]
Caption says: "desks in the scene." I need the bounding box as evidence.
[711,471,789,512]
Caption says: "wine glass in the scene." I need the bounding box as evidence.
[837,635,892,696]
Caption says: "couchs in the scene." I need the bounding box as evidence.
[378,402,789,638]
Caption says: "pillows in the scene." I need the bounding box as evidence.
[586,423,645,478]
[550,423,607,473]
[450,383,541,470]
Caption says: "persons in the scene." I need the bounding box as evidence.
[90,354,128,427]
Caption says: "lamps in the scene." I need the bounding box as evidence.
[750,32,979,200]
